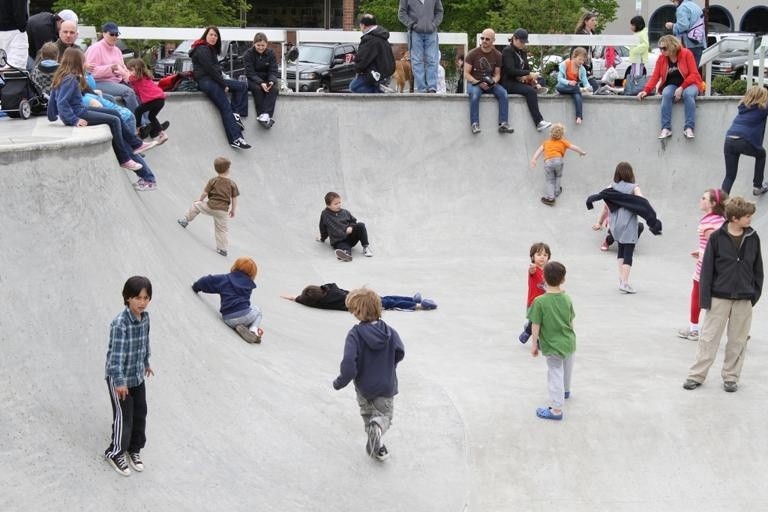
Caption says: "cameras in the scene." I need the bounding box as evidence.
[482,72,495,87]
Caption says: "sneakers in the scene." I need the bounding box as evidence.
[682,129,695,140]
[682,377,703,390]
[540,186,564,207]
[723,380,738,392]
[361,245,375,257]
[520,323,532,345]
[365,422,390,464]
[469,118,585,134]
[333,246,354,262]
[177,218,230,256]
[752,182,768,197]
[234,323,264,347]
[679,328,699,341]
[126,449,145,473]
[105,450,132,476]
[413,293,438,310]
[656,128,673,140]
[599,242,610,252]
[222,113,276,149]
[620,281,637,295]
[120,119,171,190]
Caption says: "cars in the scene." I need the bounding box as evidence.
[526,45,657,91]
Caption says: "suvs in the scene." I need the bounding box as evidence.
[153,39,254,92]
[704,30,767,92]
[276,42,359,95]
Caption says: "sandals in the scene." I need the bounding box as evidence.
[536,390,570,420]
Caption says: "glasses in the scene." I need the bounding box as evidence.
[108,32,120,37]
[481,37,491,42]
[658,46,669,51]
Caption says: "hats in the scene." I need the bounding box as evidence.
[101,22,121,33]
[515,28,529,43]
[57,8,79,26]
[535,86,548,96]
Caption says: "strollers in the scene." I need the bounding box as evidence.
[0,49,53,121]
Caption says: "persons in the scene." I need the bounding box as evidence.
[683,196,763,392]
[519,242,552,351]
[531,123,588,206]
[637,0,707,139]
[0,0,81,107]
[398,1,444,93]
[189,26,280,150]
[104,275,155,476]
[191,256,264,344]
[721,87,767,199]
[556,11,649,124]
[46,22,170,192]
[679,189,729,341]
[585,162,662,293]
[281,282,437,311]
[346,14,396,93]
[332,287,405,461]
[316,193,374,263]
[177,156,241,255]
[592,202,644,250]
[526,261,578,420]
[464,28,552,133]
